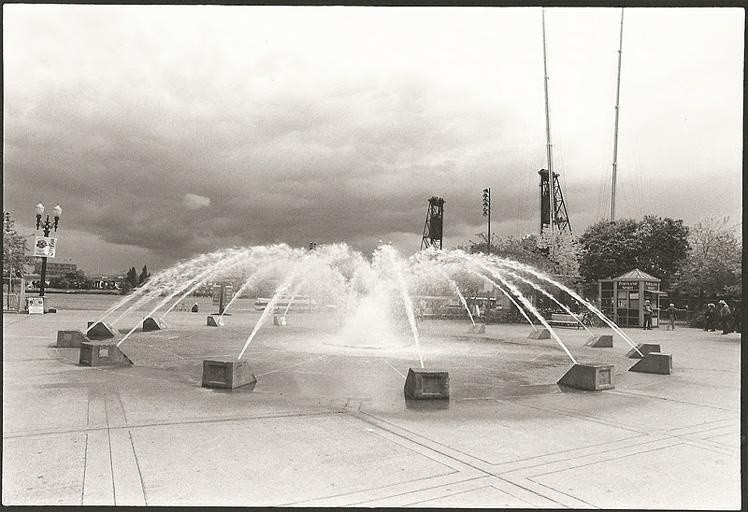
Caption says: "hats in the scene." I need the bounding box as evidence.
[718,299,726,304]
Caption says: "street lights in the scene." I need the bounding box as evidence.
[35,201,62,301]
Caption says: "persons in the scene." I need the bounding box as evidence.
[695,298,742,335]
[642,300,653,330]
[666,304,679,331]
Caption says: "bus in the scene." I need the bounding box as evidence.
[414,296,502,321]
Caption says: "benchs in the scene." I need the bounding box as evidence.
[546,314,588,330]
